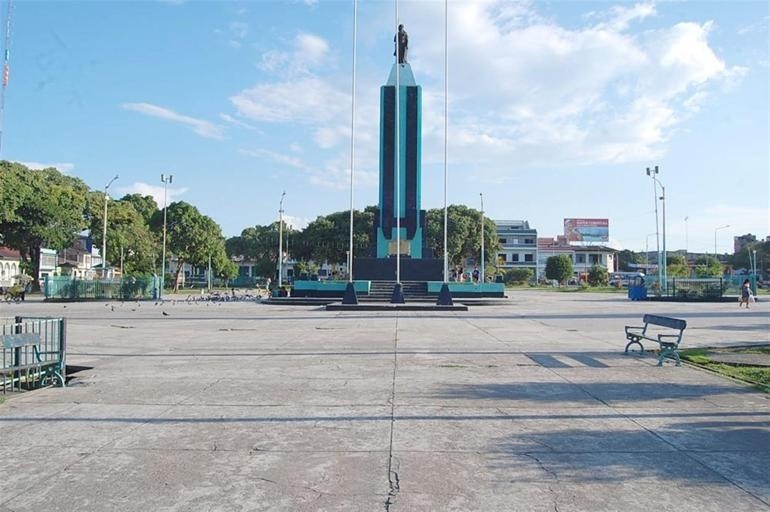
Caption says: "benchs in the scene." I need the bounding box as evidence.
[0,333,64,395]
[624,314,686,367]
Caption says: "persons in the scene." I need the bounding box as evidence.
[448,262,481,281]
[393,24,410,64]
[738,279,753,308]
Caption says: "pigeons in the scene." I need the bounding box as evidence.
[63,306,67,308]
[105,294,263,311]
[162,311,169,317]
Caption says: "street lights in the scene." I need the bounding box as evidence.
[645,165,669,293]
[278,191,287,286]
[714,224,731,257]
[478,192,485,282]
[100,175,119,278]
[159,173,174,290]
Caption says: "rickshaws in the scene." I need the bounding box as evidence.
[4,275,33,303]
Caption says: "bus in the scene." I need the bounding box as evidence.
[605,271,645,288]
